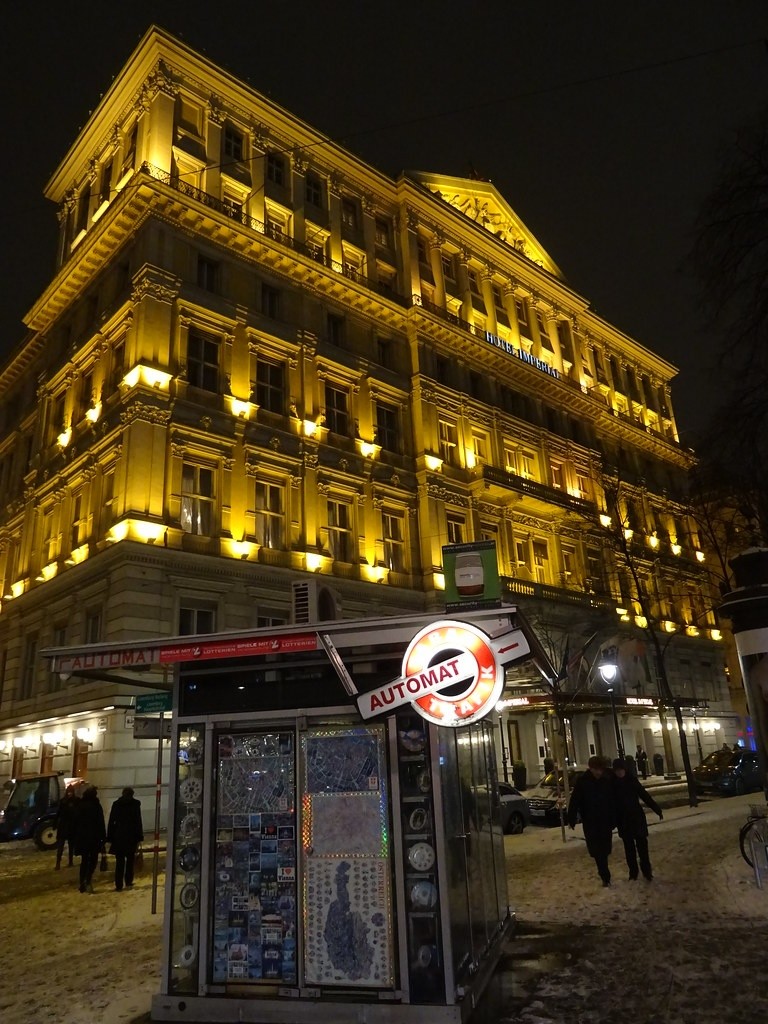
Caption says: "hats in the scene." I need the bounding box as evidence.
[66,784,74,793]
[588,756,605,770]
[611,758,628,772]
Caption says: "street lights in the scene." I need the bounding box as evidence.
[598,649,625,760]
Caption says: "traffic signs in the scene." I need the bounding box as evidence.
[135,691,172,714]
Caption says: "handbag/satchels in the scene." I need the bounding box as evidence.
[134,842,144,874]
[100,852,109,871]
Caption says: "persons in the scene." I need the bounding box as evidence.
[569,755,623,888]
[106,787,144,891]
[609,758,663,883]
[53,784,81,870]
[73,786,107,895]
[634,743,650,781]
[733,743,742,752]
[543,750,557,775]
[445,764,484,889]
[720,742,731,751]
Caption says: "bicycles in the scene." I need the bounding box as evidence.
[737,792,768,871]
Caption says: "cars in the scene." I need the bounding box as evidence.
[498,781,530,835]
[526,765,589,827]
[692,749,761,796]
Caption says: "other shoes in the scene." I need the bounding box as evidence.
[628,871,640,881]
[112,888,122,892]
[55,864,60,870]
[603,879,609,886]
[126,883,134,887]
[80,887,86,893]
[68,858,74,866]
[641,870,653,881]
